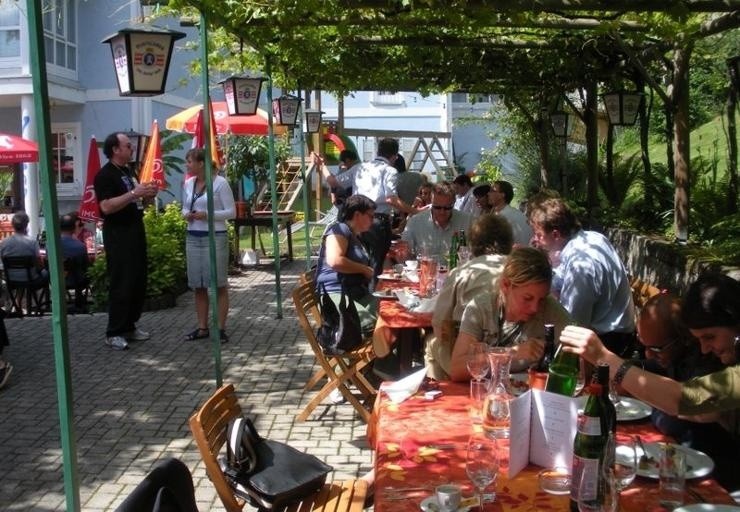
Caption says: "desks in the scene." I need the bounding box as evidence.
[229,212,297,267]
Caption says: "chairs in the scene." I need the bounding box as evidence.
[41,257,93,321]
[115,459,197,510]
[627,274,666,313]
[189,382,368,510]
[302,268,326,327]
[290,280,374,425]
[1,247,44,321]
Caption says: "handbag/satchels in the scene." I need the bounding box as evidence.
[218,417,332,512]
[317,278,362,355]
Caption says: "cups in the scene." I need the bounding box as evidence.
[415,254,440,297]
[577,460,619,512]
[468,375,489,424]
[658,444,686,509]
[466,342,489,382]
[435,484,460,510]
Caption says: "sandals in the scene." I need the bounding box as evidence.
[220,329,228,344]
[184,328,209,341]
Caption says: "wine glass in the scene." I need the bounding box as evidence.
[464,428,498,512]
[601,437,644,510]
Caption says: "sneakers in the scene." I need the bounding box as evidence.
[105,329,149,350]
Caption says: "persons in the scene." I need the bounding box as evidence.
[180,149,238,344]
[560,276,739,492]
[309,139,634,382]
[94,132,159,351]
[0,211,105,392]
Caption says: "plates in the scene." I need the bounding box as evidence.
[671,504,740,512]
[570,393,652,425]
[403,266,447,272]
[420,496,471,512]
[629,443,718,480]
[508,372,530,396]
[372,288,418,299]
[377,273,402,280]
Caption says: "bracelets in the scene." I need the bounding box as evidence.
[131,190,137,199]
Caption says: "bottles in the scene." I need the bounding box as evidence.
[495,322,524,347]
[542,323,583,396]
[449,229,468,270]
[591,363,618,473]
[480,346,521,443]
[571,381,611,512]
[536,325,560,374]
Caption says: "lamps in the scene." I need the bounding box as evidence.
[303,80,326,138]
[271,60,307,129]
[548,104,578,140]
[216,37,269,119]
[600,74,648,128]
[102,2,190,99]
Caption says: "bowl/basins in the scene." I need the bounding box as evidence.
[536,467,571,494]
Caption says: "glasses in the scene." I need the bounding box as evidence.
[649,336,679,352]
[432,206,452,210]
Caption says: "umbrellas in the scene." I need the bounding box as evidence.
[166,101,287,137]
[138,120,166,216]
[185,96,226,184]
[0,130,40,164]
[77,137,103,247]
